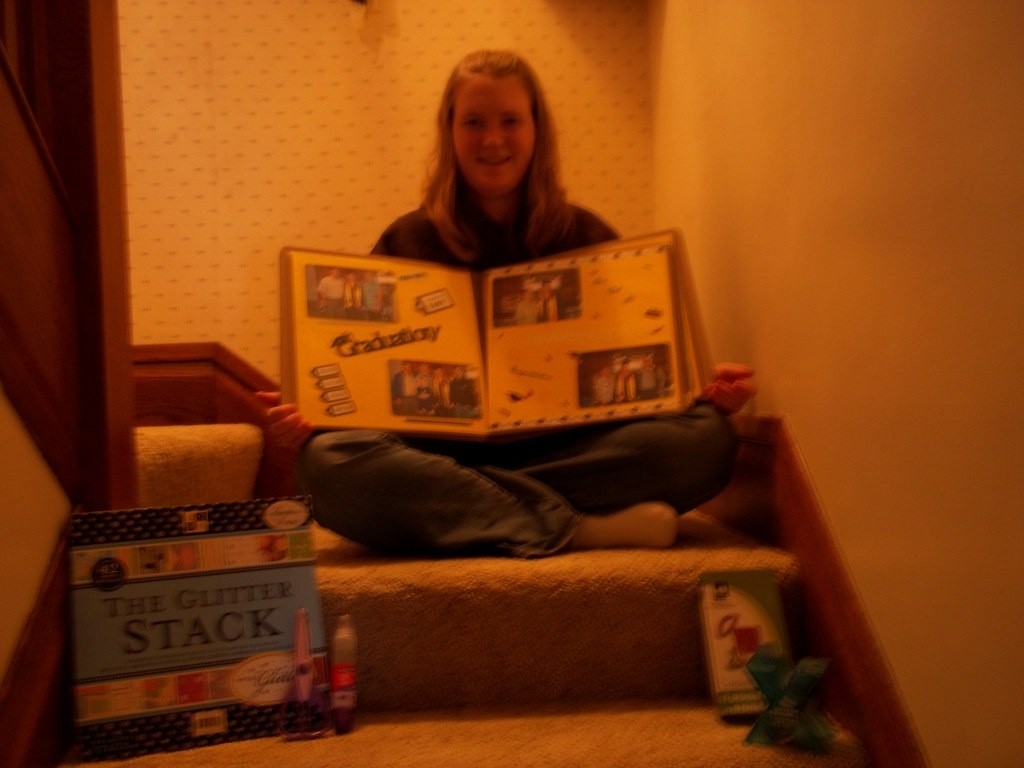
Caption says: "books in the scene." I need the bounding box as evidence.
[697,568,795,719]
[279,228,709,438]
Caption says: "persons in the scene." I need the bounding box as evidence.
[255,50,756,559]
[514,280,557,324]
[594,357,665,406]
[392,361,475,419]
[316,268,383,321]
